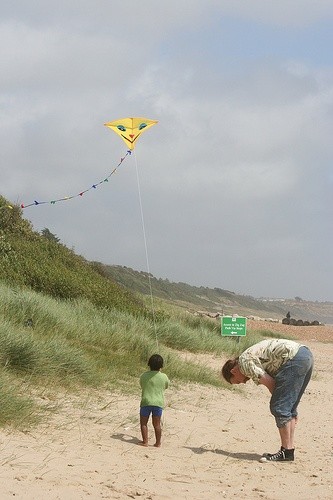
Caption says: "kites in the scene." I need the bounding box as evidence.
[2,118,160,213]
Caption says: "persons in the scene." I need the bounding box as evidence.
[221,338,314,462]
[139,354,169,447]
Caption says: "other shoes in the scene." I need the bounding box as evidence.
[260,447,295,462]
